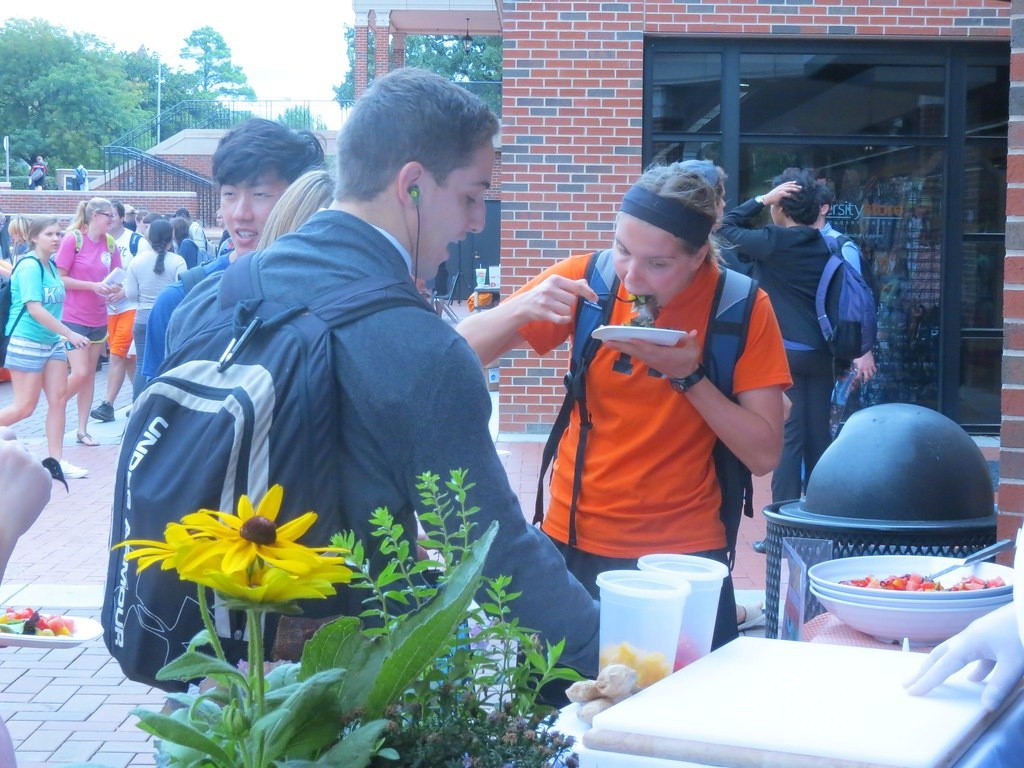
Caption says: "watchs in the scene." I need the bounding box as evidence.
[670,363,705,393]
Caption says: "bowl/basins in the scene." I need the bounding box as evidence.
[807,556,1015,647]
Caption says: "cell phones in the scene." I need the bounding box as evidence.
[65,341,85,350]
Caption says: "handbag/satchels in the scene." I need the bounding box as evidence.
[31,168,43,181]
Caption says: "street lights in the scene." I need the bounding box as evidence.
[155,63,165,145]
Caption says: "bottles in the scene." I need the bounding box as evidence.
[830,361,858,406]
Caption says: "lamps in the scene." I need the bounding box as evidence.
[462,18,473,54]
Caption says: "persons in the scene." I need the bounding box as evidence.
[452,168,793,710]
[903,601,1024,711]
[31,156,46,190]
[75,165,89,190]
[164,70,601,704]
[141,119,336,380]
[0,426,53,768]
[0,198,234,479]
[681,159,877,629]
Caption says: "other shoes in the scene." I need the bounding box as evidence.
[57,459,91,478]
[752,539,766,555]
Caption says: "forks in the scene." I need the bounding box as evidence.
[582,293,639,302]
[41,457,69,494]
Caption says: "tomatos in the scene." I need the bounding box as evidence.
[866,572,1006,591]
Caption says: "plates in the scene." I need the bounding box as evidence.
[591,326,689,346]
[0,616,103,648]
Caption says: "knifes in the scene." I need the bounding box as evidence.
[927,539,1013,580]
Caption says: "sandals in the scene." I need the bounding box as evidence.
[76,433,100,447]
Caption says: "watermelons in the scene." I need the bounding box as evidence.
[7,608,74,632]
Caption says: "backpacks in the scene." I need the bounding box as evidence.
[522,244,755,569]
[816,258,889,383]
[0,256,58,368]
[76,167,87,185]
[100,245,434,690]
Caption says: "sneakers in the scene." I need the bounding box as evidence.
[90,399,116,422]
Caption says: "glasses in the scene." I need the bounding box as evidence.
[99,212,121,220]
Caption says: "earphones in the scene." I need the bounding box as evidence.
[408,185,420,205]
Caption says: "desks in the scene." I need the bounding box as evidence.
[474,285,500,314]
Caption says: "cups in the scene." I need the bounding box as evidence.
[475,269,486,285]
[637,553,729,673]
[595,570,692,704]
